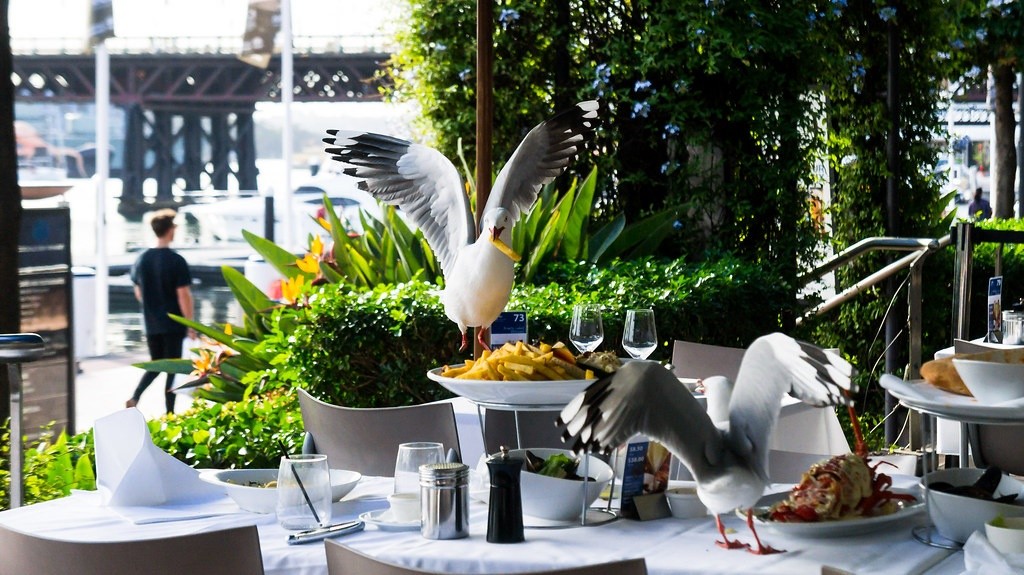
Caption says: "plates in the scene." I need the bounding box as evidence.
[917,467,1024,541]
[736,483,929,537]
[357,510,423,532]
[887,380,1024,426]
[426,363,609,407]
[215,468,361,514]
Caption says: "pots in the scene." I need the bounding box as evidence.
[1000,302,1024,346]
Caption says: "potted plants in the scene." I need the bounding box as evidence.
[287,274,472,479]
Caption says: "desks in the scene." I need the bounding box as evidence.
[425,393,805,468]
[0,472,1024,575]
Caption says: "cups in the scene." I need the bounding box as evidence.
[275,455,333,530]
[393,443,444,500]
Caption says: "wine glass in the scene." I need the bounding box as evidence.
[622,309,659,361]
[569,303,604,358]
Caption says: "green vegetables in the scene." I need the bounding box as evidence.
[534,451,579,480]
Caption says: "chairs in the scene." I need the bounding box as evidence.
[0,337,848,575]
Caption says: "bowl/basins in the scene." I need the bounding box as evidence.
[485,447,614,521]
[982,514,1024,569]
[386,493,422,515]
[952,358,1024,403]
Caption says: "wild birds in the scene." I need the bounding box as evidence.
[320,97,600,356]
[554,332,860,555]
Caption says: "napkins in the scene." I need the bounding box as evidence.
[48,407,239,528]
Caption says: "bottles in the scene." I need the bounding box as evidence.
[483,444,525,543]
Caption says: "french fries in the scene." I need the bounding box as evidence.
[441,341,594,384]
[487,235,522,261]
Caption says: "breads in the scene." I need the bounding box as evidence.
[919,346,1024,397]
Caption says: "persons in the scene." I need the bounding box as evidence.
[969,187,992,221]
[125,213,196,414]
